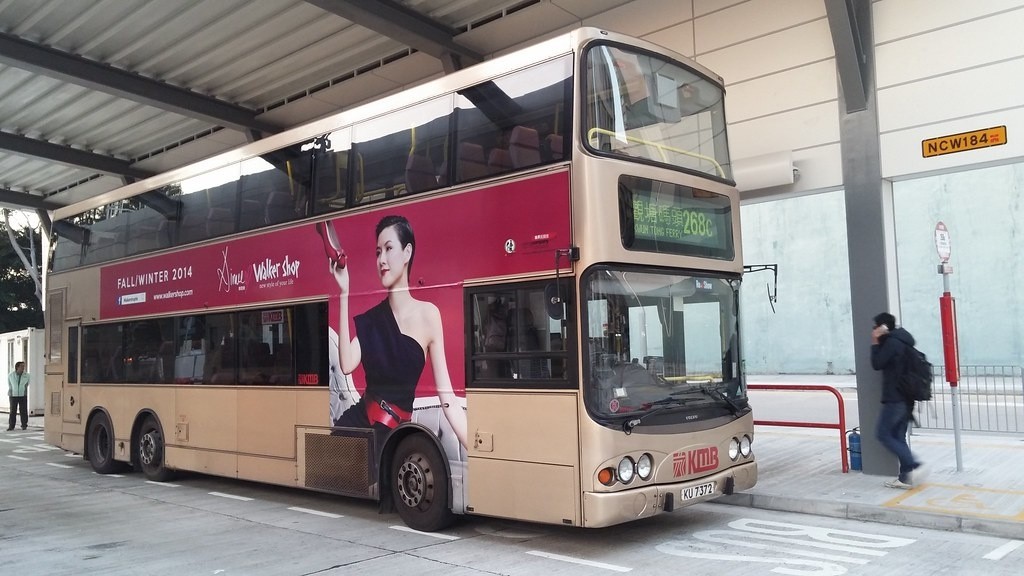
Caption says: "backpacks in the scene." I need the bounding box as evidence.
[906,346,932,401]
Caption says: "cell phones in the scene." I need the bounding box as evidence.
[876,325,888,335]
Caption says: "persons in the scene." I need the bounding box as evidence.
[7,361,31,432]
[328,213,469,453]
[868,311,937,490]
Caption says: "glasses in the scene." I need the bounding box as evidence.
[873,326,878,329]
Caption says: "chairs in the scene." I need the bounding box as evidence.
[86,124,563,270]
[84,331,293,387]
[503,308,550,379]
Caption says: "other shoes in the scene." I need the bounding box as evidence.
[911,463,925,478]
[316,220,346,268]
[22,426,26,430]
[884,479,912,490]
[7,428,14,431]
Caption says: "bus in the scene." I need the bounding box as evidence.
[43,25,780,531]
